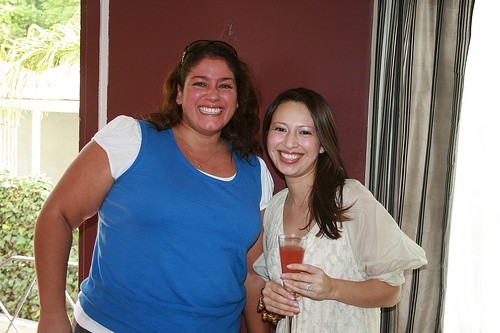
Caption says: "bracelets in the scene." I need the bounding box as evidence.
[257,288,286,325]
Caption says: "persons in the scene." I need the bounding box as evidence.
[34,39,274,333]
[252,87,428,333]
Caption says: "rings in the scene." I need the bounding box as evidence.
[307,284,312,290]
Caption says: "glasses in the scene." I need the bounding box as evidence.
[181,40,239,65]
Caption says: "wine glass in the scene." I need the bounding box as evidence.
[279,232,308,333]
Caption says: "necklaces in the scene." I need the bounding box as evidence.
[182,135,222,167]
